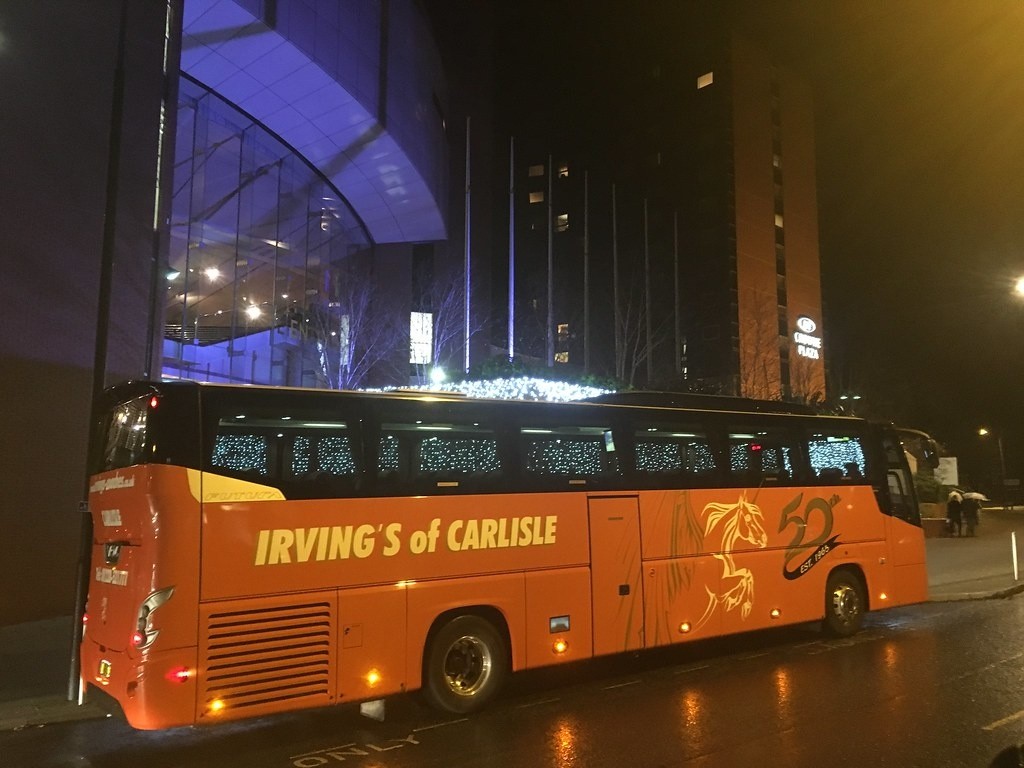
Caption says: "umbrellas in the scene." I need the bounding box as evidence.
[962,493,991,501]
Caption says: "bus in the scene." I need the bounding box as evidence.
[80,381,939,730]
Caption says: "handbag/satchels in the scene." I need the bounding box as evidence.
[946,519,954,533]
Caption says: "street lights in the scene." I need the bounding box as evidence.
[978,427,1006,481]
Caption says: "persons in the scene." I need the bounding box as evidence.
[946,494,963,539]
[961,498,982,538]
